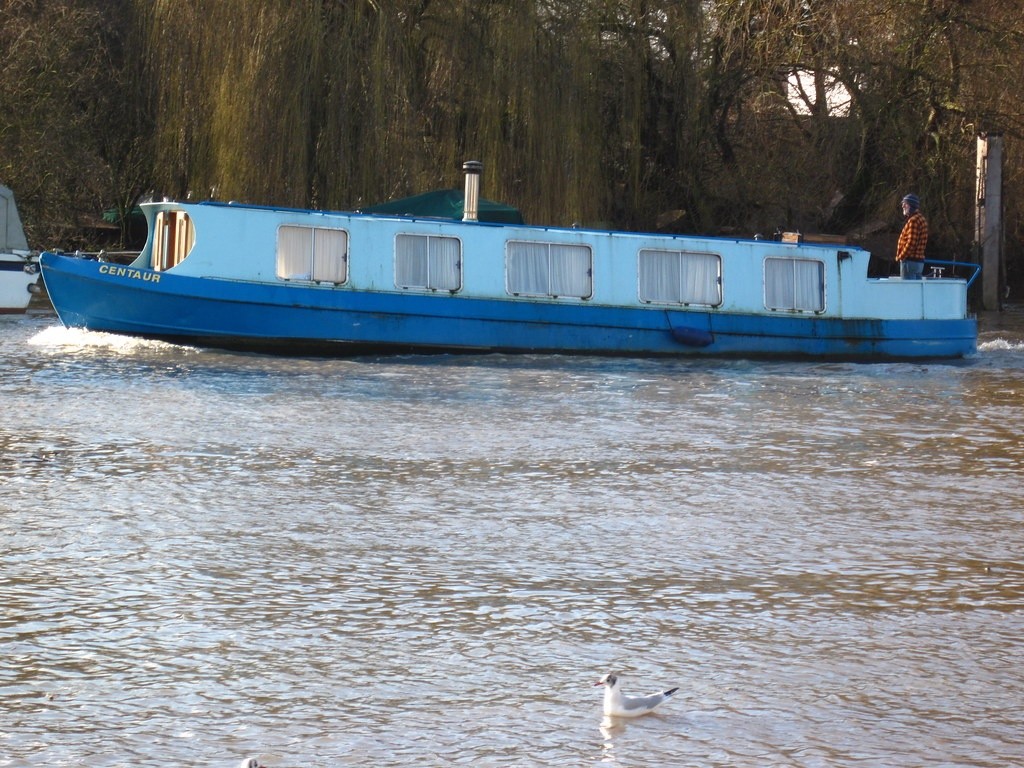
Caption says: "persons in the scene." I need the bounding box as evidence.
[895,194,928,280]
[97,249,114,263]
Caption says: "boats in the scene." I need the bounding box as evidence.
[0,185,45,313]
[38,162,983,361]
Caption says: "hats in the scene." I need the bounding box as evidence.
[903,194,920,209]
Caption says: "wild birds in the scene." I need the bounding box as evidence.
[594,674,680,717]
